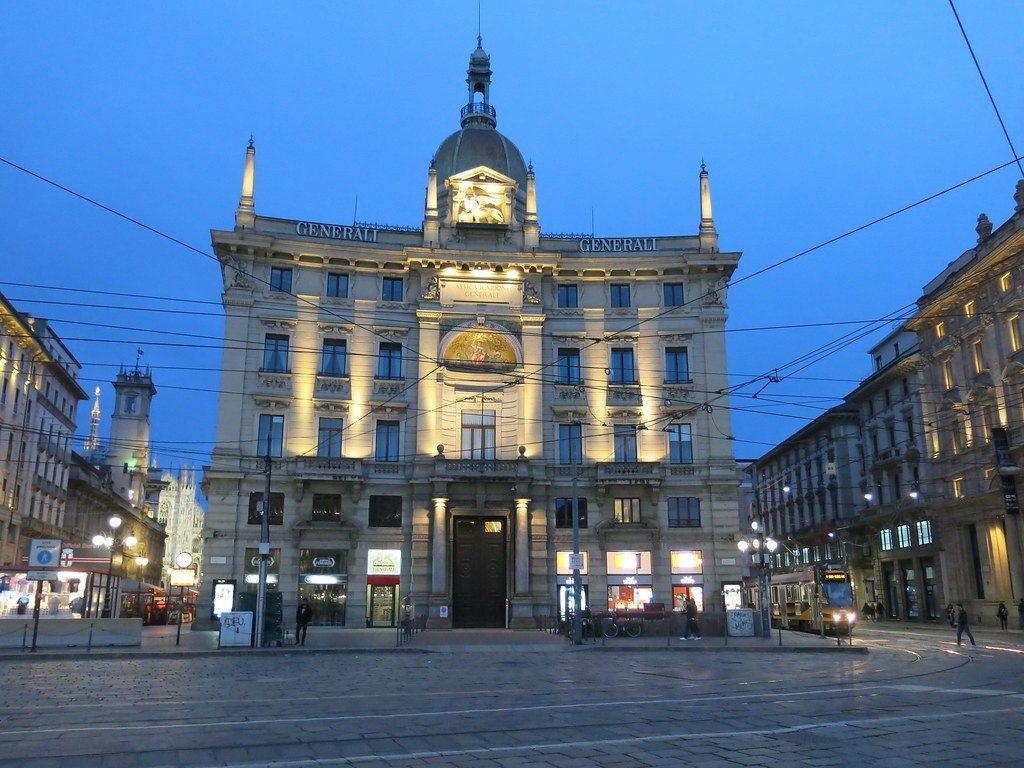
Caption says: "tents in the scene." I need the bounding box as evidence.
[119,578,166,594]
[155,586,199,605]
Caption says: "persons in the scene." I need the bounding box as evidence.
[1017,598,1024,631]
[0,573,86,616]
[470,339,484,364]
[997,602,1008,630]
[679,597,699,641]
[295,597,313,646]
[955,602,976,647]
[945,604,955,628]
[688,598,702,639]
[861,602,886,623]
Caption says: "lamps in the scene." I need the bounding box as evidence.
[517,446,528,460]
[434,444,445,458]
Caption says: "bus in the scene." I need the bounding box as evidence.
[743,564,856,635]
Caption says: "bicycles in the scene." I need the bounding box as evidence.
[602,612,642,637]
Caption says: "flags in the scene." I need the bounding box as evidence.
[138,348,143,355]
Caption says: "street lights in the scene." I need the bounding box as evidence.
[90,514,138,617]
[737,518,778,636]
[134,550,149,619]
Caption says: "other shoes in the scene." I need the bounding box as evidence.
[694,637,701,640]
[680,637,687,640]
[688,636,694,639]
